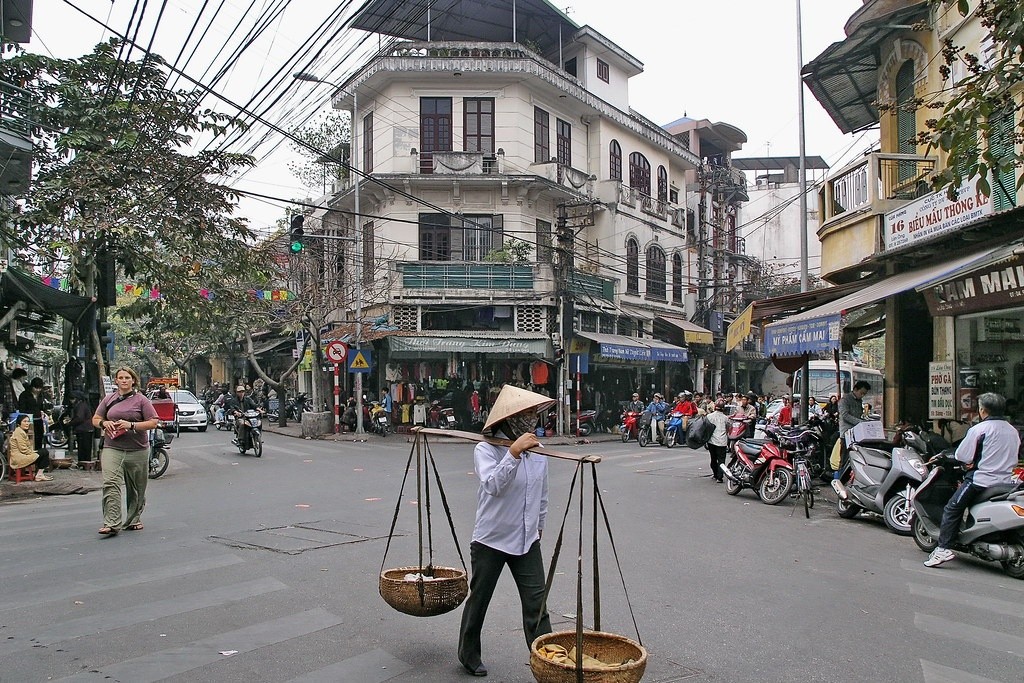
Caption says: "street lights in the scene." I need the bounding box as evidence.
[293,70,365,435]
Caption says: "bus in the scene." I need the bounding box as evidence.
[792,360,883,415]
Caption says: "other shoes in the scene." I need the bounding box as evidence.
[237,442,243,445]
[213,421,219,425]
[467,662,487,676]
[717,479,723,483]
[35,475,53,482]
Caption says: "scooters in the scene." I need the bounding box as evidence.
[146,427,175,479]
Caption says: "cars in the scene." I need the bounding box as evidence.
[764,398,800,418]
[149,388,208,433]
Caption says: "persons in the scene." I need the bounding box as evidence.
[141,384,179,401]
[0,361,53,451]
[62,390,92,470]
[332,378,1023,573]
[8,415,54,482]
[91,367,159,533]
[197,381,265,447]
[457,383,557,677]
[705,398,732,483]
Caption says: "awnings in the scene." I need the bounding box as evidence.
[658,317,713,345]
[764,243,1016,356]
[726,278,895,354]
[575,331,688,362]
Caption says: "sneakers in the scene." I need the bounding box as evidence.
[924,547,955,566]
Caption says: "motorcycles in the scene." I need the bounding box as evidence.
[212,403,265,458]
[0,419,18,480]
[727,411,753,454]
[719,416,821,505]
[267,390,308,422]
[544,409,597,436]
[429,400,458,430]
[621,406,688,449]
[362,395,391,437]
[831,425,1024,579]
[45,405,72,447]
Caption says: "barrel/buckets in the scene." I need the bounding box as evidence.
[546,430,553,436]
[960,367,980,421]
[8,412,24,431]
[537,428,544,436]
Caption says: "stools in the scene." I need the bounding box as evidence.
[397,426,405,434]
[406,426,415,435]
[8,466,34,485]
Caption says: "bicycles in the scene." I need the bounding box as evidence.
[778,431,819,519]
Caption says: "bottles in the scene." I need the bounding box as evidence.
[985,317,1021,339]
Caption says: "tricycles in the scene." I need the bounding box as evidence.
[150,398,180,439]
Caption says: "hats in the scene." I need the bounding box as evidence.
[237,386,245,392]
[482,384,558,435]
[632,393,639,396]
[654,393,661,398]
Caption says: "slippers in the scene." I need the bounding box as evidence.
[99,527,118,534]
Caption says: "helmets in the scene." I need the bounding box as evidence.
[782,395,789,399]
[678,392,685,397]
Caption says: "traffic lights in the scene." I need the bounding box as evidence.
[97,319,112,348]
[289,212,304,253]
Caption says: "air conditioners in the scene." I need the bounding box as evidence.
[757,178,768,186]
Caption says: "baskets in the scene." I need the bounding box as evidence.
[379,566,468,617]
[528,630,647,683]
[639,418,650,425]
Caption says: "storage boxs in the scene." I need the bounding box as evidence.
[844,421,885,449]
